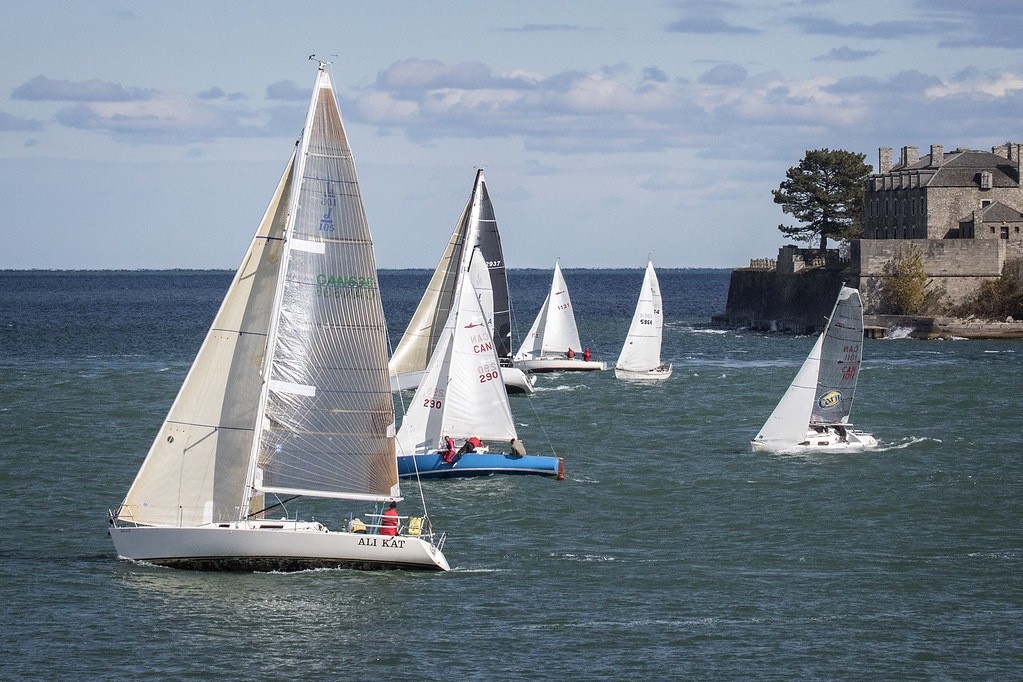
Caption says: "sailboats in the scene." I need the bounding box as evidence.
[744,275,880,453]
[512,258,605,371]
[97,50,459,573]
[612,249,674,383]
[393,268,565,483]
[384,165,537,395]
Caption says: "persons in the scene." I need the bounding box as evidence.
[499,359,506,367]
[351,518,367,534]
[443,436,457,468]
[509,438,526,458]
[582,347,591,362]
[505,359,513,367]
[452,436,483,468]
[376,500,400,537]
[567,348,575,357]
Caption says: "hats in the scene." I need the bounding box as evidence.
[389,501,396,508]
